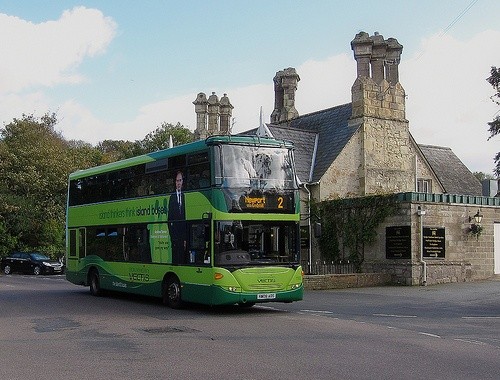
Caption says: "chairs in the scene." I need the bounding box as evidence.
[248,246,263,259]
[150,174,211,194]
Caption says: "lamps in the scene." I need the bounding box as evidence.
[469,209,483,223]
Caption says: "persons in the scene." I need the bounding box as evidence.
[166,171,189,265]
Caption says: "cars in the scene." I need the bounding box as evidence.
[2,251,62,278]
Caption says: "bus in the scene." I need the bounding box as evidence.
[65,132,303,312]
